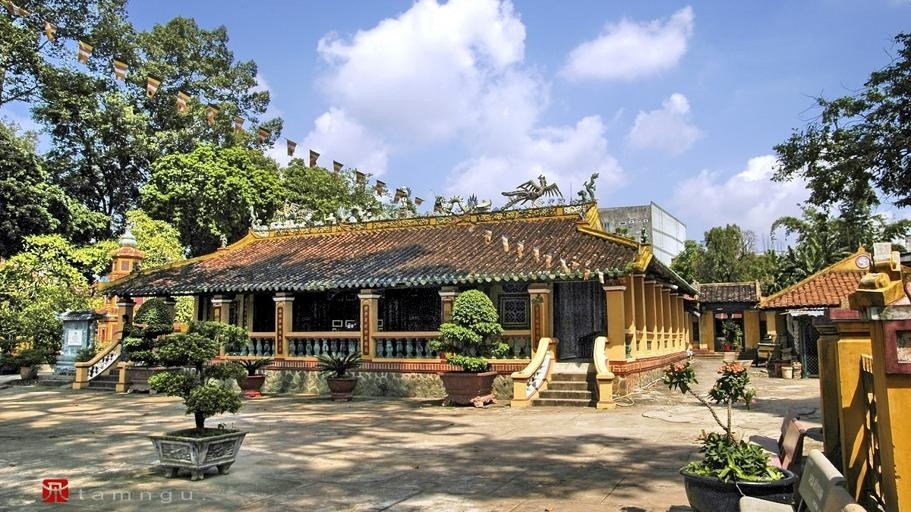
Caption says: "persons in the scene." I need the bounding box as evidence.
[686,343,694,358]
[725,323,735,344]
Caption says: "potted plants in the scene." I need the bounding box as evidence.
[314,351,364,402]
[429,290,510,408]
[18,349,44,380]
[122,299,183,395]
[231,358,274,398]
[147,319,248,482]
[662,360,800,512]
